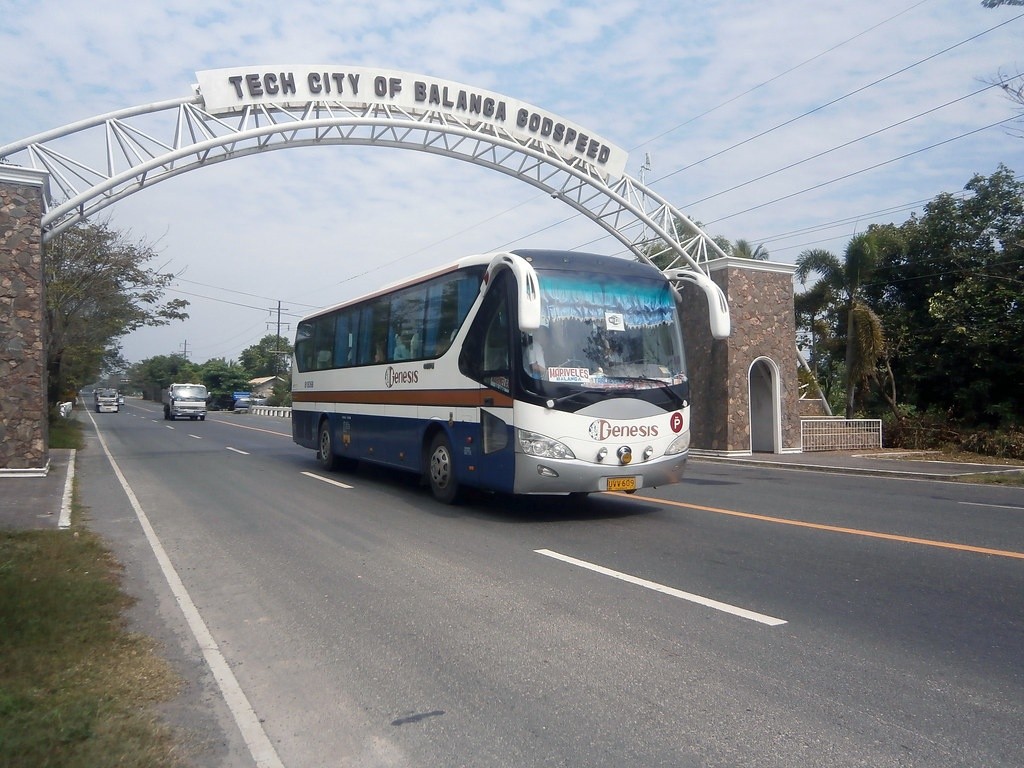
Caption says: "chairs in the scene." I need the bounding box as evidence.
[317,350,333,369]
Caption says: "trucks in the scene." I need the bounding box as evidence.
[162,383,210,420]
[95,386,121,413]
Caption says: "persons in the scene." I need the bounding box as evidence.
[347,326,416,367]
[524,325,548,379]
[604,343,623,367]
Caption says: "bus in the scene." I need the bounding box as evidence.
[292,248,732,505]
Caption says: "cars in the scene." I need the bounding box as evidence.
[118,394,125,406]
